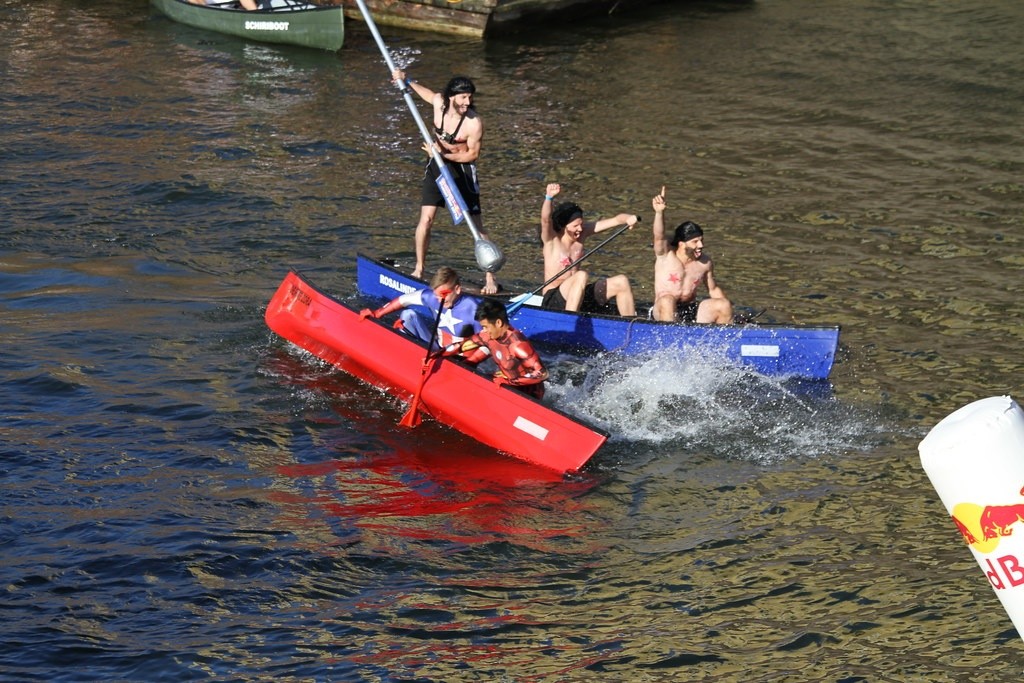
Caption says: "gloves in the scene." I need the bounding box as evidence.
[493,376,510,388]
[461,348,488,367]
[359,296,404,321]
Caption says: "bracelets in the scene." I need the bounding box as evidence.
[405,78,411,85]
[546,195,553,200]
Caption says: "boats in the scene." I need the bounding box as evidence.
[265,267,610,481]
[353,251,842,381]
[151,0,345,51]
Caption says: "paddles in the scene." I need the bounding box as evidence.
[395,293,444,427]
[502,216,644,314]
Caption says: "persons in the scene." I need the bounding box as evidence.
[422,299,549,399]
[652,186,734,325]
[189,0,257,10]
[359,266,483,369]
[540,183,637,317]
[390,70,498,294]
